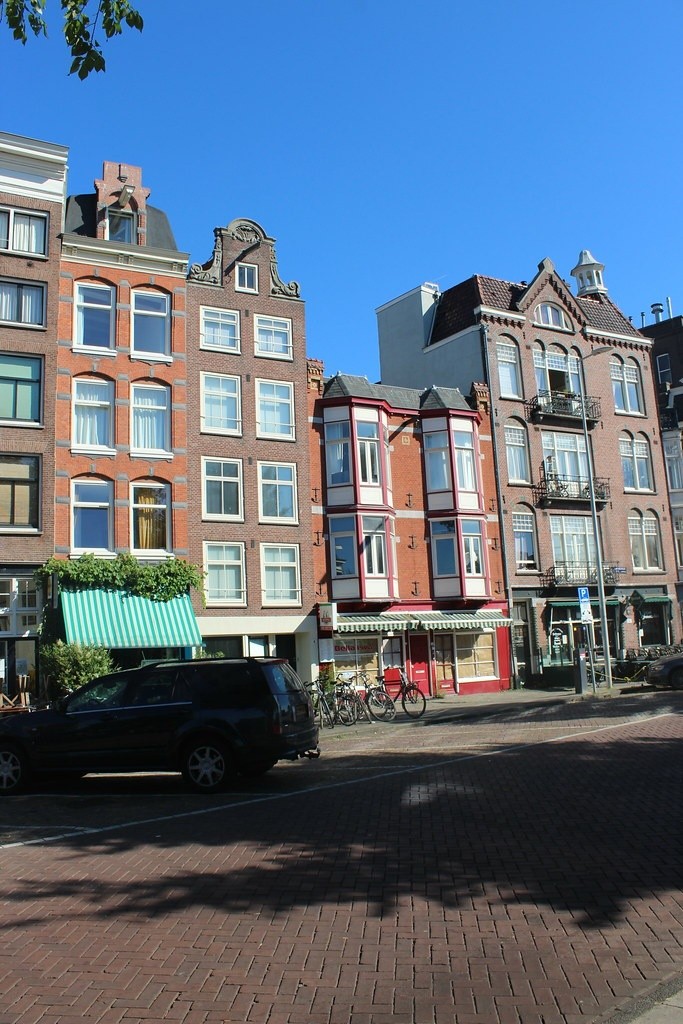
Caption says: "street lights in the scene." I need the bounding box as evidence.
[582,346,616,687]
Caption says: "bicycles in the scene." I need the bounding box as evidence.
[305,665,426,729]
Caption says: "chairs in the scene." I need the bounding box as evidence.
[553,477,570,497]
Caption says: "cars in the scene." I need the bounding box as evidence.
[645,652,683,690]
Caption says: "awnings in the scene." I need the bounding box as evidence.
[61,586,205,649]
[547,596,619,607]
[411,609,514,630]
[643,594,670,603]
[337,611,420,633]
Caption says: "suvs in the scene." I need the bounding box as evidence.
[1,656,319,795]
